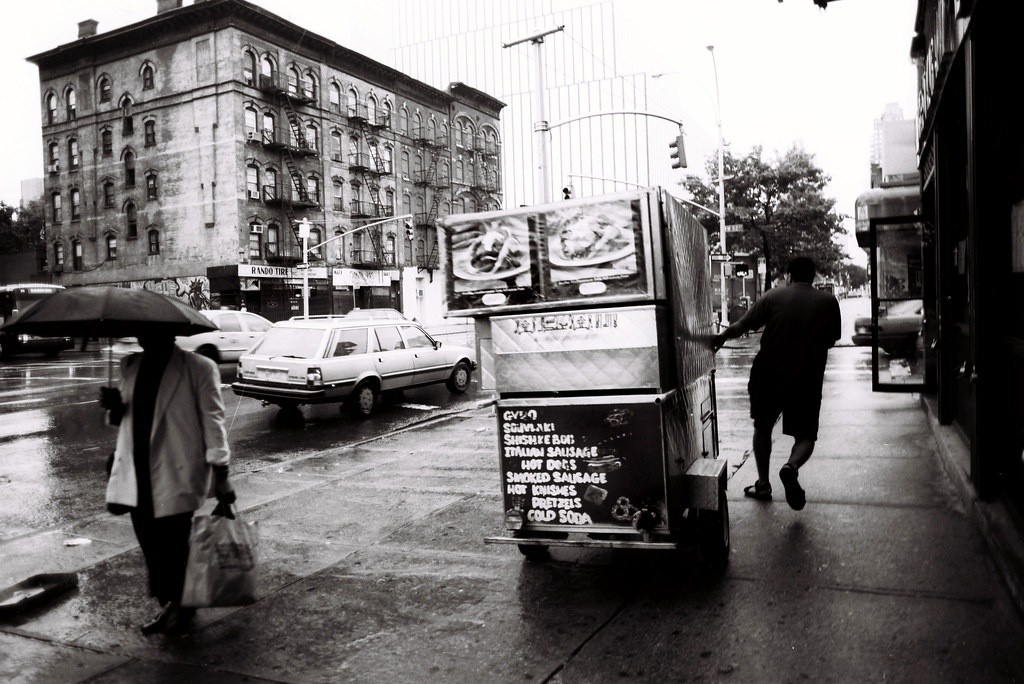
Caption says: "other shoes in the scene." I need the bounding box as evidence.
[140,598,196,636]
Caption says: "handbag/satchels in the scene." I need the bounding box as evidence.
[181,498,257,608]
[105,450,131,515]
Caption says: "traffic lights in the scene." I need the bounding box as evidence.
[404,221,415,242]
[669,133,690,171]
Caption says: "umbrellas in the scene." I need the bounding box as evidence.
[12,278,216,397]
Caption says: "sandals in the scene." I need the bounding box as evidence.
[780,463,807,510]
[744,480,772,501]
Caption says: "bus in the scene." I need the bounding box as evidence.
[0,282,75,359]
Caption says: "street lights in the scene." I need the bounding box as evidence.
[707,43,730,336]
[562,185,574,200]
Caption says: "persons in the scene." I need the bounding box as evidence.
[715,249,844,513]
[100,321,235,636]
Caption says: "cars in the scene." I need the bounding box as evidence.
[231,307,477,417]
[176,309,275,365]
[852,297,925,362]
